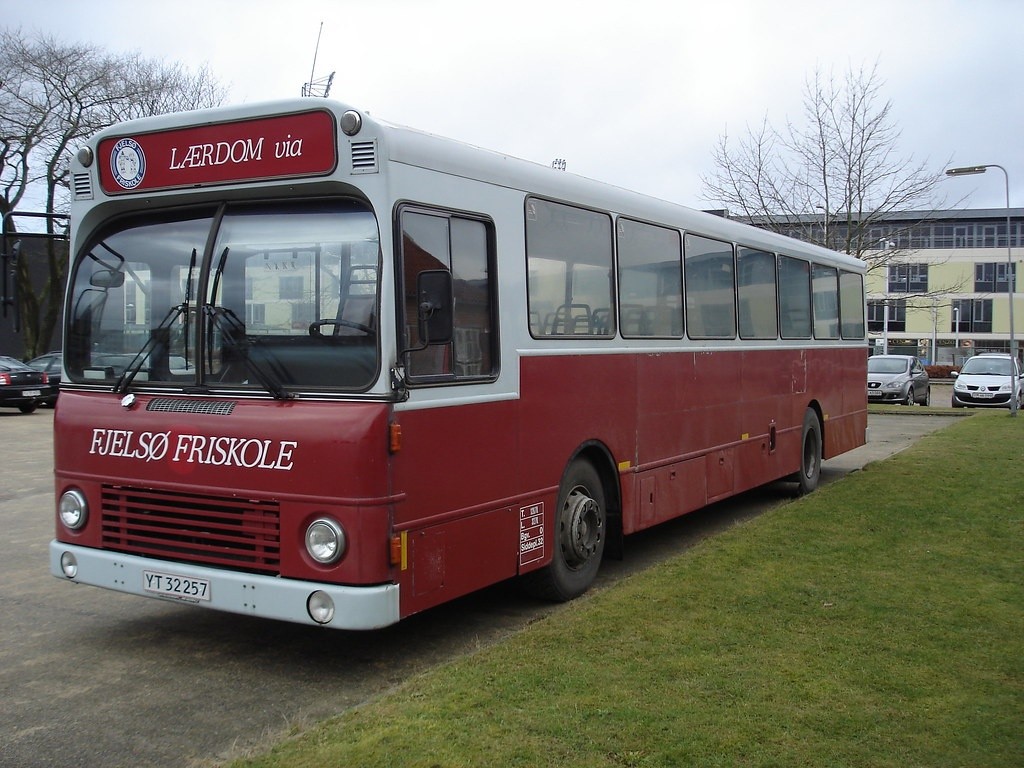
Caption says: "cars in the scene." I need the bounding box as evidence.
[0,356,53,414]
[950,352,1024,411]
[867,355,931,407]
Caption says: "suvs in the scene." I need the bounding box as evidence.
[25,349,104,408]
[90,352,197,369]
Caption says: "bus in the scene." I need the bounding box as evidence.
[0,98,869,633]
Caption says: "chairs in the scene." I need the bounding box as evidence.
[332,265,378,336]
[603,304,646,335]
[550,303,594,335]
[785,308,811,337]
[543,312,573,335]
[638,306,675,337]
[573,315,602,335]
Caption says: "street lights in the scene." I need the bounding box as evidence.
[879,237,895,356]
[944,164,1018,417]
[953,308,959,348]
[123,277,135,355]
